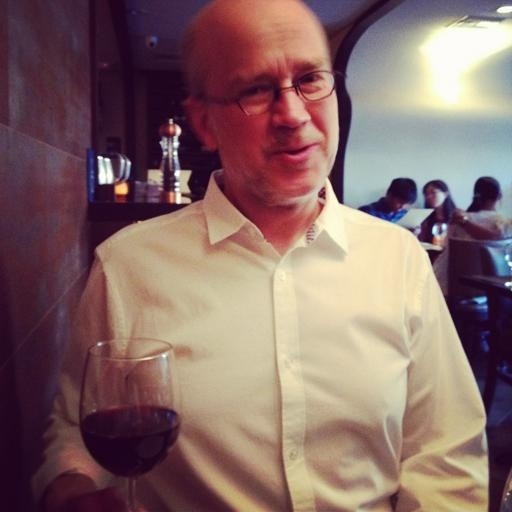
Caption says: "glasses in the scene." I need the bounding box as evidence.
[195,69,343,117]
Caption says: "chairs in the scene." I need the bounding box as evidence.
[447,227,510,423]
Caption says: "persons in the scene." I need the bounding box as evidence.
[446,176,511,241]
[27,0,489,512]
[358,178,418,237]
[418,179,463,242]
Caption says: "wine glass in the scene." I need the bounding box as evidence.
[80,337,180,512]
[503,242,512,289]
